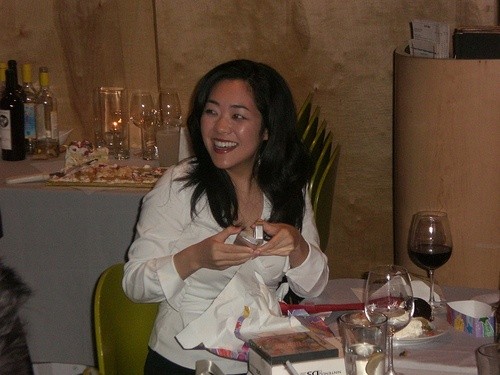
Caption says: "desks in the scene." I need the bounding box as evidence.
[0,151,161,366]
[296,278,500,375]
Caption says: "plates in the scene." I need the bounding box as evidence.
[393,316,451,345]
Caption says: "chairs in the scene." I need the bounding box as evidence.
[93,262,160,375]
[295,91,343,255]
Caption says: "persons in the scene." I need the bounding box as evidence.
[121,58,329,375]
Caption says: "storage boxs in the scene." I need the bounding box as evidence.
[247,337,386,375]
[233,225,264,250]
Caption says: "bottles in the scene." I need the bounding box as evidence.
[0,59,60,161]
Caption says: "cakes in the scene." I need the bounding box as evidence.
[65,140,127,180]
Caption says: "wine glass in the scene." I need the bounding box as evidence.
[364,264,415,375]
[92,89,182,160]
[406,211,453,314]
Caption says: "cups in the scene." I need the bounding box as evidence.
[477,344,500,375]
[339,310,388,375]
